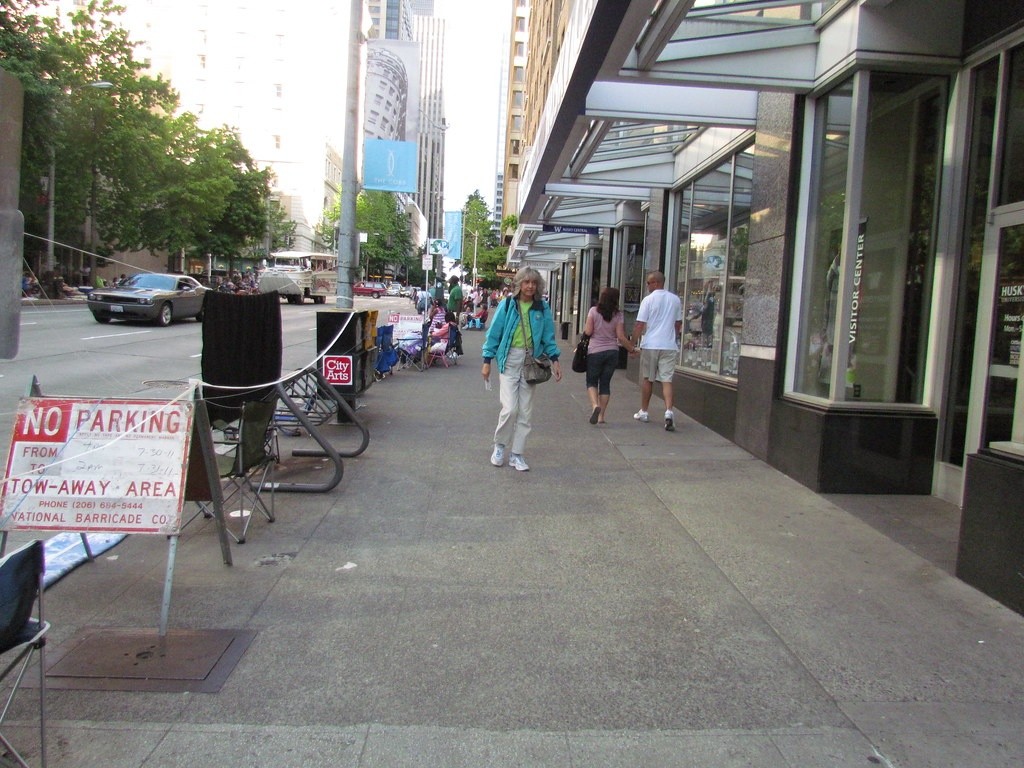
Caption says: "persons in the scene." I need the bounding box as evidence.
[102,274,126,288]
[227,274,252,291]
[415,285,433,315]
[22,271,40,296]
[629,270,682,431]
[448,275,463,333]
[427,299,457,364]
[461,287,514,330]
[482,266,562,471]
[80,262,91,286]
[584,287,635,424]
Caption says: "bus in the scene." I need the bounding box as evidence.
[259,251,338,304]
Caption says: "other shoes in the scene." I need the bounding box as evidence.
[462,325,469,329]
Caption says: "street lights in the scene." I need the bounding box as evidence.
[44,81,114,272]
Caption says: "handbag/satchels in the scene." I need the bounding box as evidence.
[455,332,463,356]
[572,336,588,372]
[524,351,552,384]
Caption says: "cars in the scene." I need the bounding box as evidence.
[86,273,214,327]
[352,281,412,299]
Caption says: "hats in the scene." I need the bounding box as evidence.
[450,275,459,283]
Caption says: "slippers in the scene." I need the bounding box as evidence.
[598,422,605,423]
[590,407,601,423]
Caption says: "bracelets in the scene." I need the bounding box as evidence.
[630,347,634,353]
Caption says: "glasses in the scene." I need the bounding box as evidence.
[647,281,655,284]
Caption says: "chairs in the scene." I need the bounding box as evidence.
[0,538,51,768]
[392,322,458,371]
[163,401,280,544]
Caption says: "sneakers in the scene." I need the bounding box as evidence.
[491,443,504,466]
[634,409,648,422]
[509,452,529,471]
[664,410,675,430]
